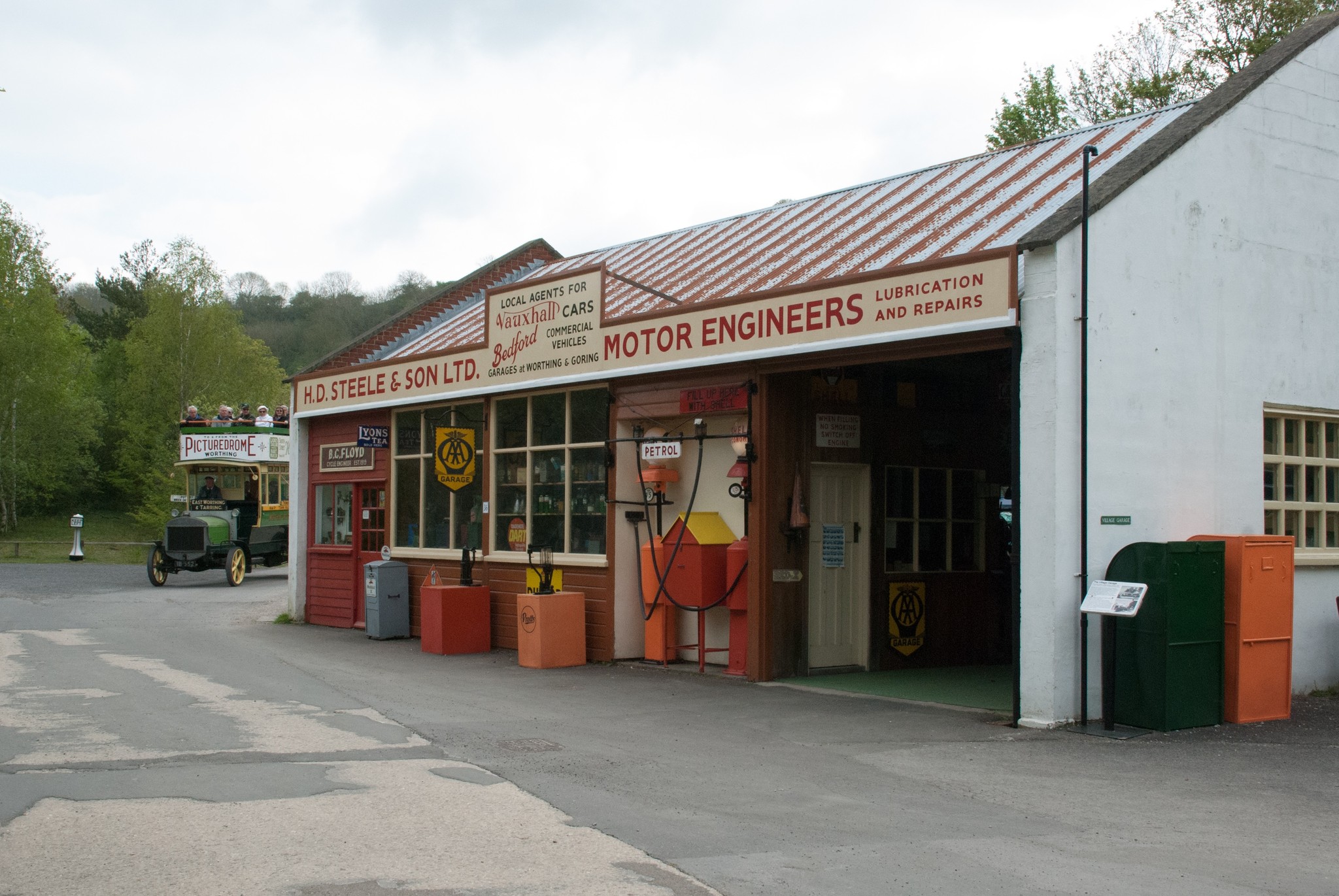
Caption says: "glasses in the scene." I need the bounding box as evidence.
[259,410,266,413]
[276,410,282,412]
[242,408,249,411]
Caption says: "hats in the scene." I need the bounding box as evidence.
[241,404,250,408]
[204,476,215,480]
[257,406,269,412]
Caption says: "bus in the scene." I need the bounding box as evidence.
[147,420,290,588]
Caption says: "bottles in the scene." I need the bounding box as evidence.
[538,494,558,514]
[574,459,606,482]
[512,494,527,514]
[571,487,607,515]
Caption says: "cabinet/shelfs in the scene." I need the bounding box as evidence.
[495,481,607,518]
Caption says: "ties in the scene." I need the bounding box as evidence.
[208,489,211,498]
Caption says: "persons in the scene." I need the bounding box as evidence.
[244,481,284,503]
[179,403,289,435]
[197,476,223,500]
